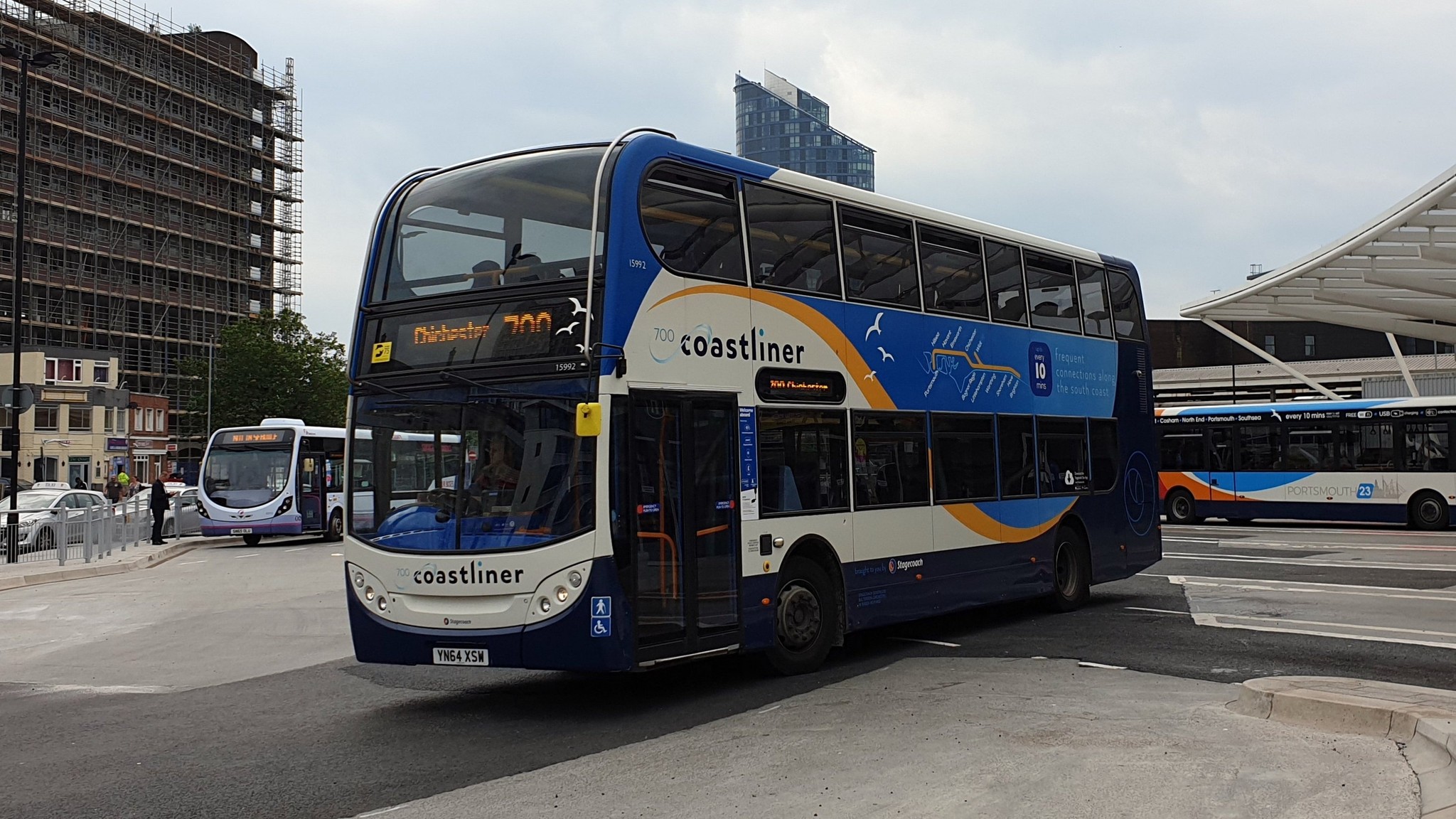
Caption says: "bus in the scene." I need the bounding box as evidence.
[1153,396,1456,532]
[196,418,469,546]
[341,126,1164,676]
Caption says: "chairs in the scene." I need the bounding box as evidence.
[471,236,1135,338]
[760,462,803,513]
[875,462,905,504]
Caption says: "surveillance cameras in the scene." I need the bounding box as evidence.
[133,443,138,447]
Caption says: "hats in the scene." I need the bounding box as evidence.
[110,474,116,479]
[121,470,125,472]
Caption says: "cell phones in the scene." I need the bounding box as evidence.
[174,491,178,494]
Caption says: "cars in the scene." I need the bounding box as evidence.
[0,476,202,556]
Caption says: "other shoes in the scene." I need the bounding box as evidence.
[159,539,168,544]
[152,540,162,545]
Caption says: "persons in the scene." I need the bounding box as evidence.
[74,477,88,490]
[150,471,175,544]
[474,434,519,514]
[104,469,142,508]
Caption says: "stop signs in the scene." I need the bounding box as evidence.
[468,451,477,460]
